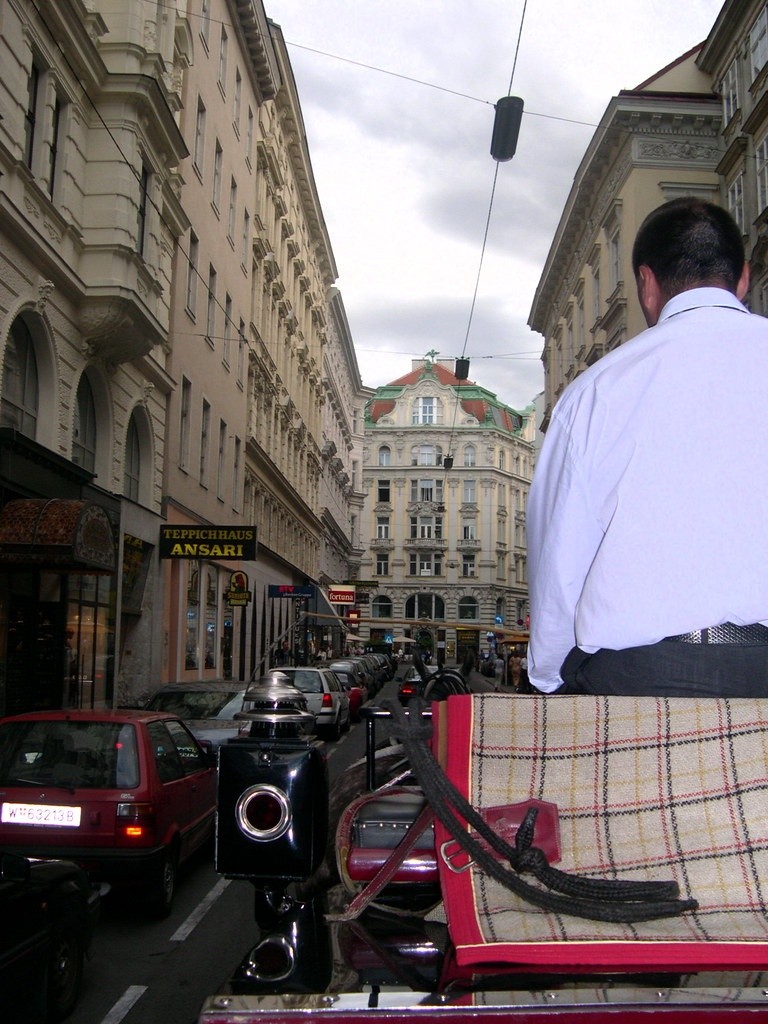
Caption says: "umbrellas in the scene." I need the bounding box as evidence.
[347,635,416,654]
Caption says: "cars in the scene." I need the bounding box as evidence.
[259,644,504,738]
[0,847,100,1024]
[138,680,260,769]
[0,710,219,918]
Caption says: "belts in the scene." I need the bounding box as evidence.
[666,623,768,648]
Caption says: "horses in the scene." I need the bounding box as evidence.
[324,649,476,874]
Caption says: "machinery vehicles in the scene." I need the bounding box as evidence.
[195,650,768,1024]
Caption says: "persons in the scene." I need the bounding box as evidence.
[527,197,768,699]
[273,640,527,689]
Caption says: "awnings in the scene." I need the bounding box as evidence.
[315,585,344,627]
